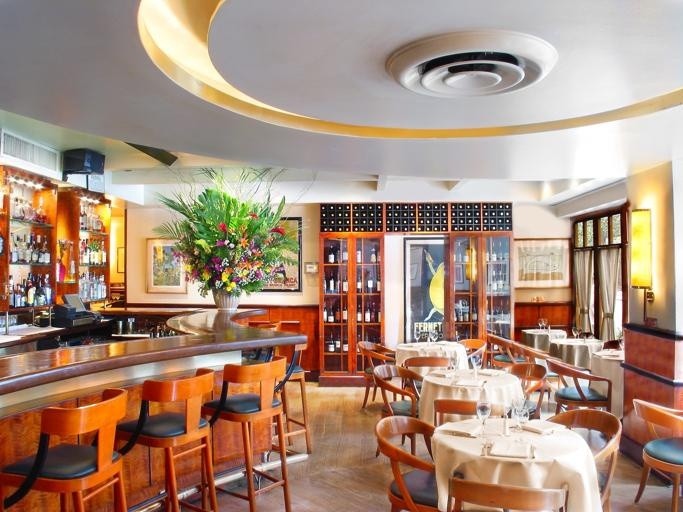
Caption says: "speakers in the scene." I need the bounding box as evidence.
[63,149,105,175]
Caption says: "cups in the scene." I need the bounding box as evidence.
[0,315,17,329]
[114,318,176,338]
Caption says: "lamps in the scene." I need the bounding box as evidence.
[628,206,658,327]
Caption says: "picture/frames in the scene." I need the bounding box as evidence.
[402,236,462,344]
[513,237,573,291]
[145,237,188,294]
[116,246,124,273]
[454,264,464,286]
[252,216,302,293]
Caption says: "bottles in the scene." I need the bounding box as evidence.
[324,238,382,355]
[7,186,113,309]
[455,238,509,341]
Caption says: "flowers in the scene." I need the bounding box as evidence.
[149,161,323,298]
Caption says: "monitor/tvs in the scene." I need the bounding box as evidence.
[62,294,87,312]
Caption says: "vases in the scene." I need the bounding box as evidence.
[211,288,240,312]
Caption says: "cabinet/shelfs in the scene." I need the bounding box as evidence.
[443,231,516,349]
[31,182,111,313]
[0,163,56,313]
[319,202,514,234]
[318,231,385,389]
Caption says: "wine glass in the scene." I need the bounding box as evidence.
[534,318,622,361]
[413,326,566,458]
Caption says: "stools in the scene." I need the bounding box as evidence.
[2,387,128,511]
[116,368,219,510]
[243,323,280,363]
[264,343,313,456]
[201,356,292,510]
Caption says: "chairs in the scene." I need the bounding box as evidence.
[359,324,682,511]
